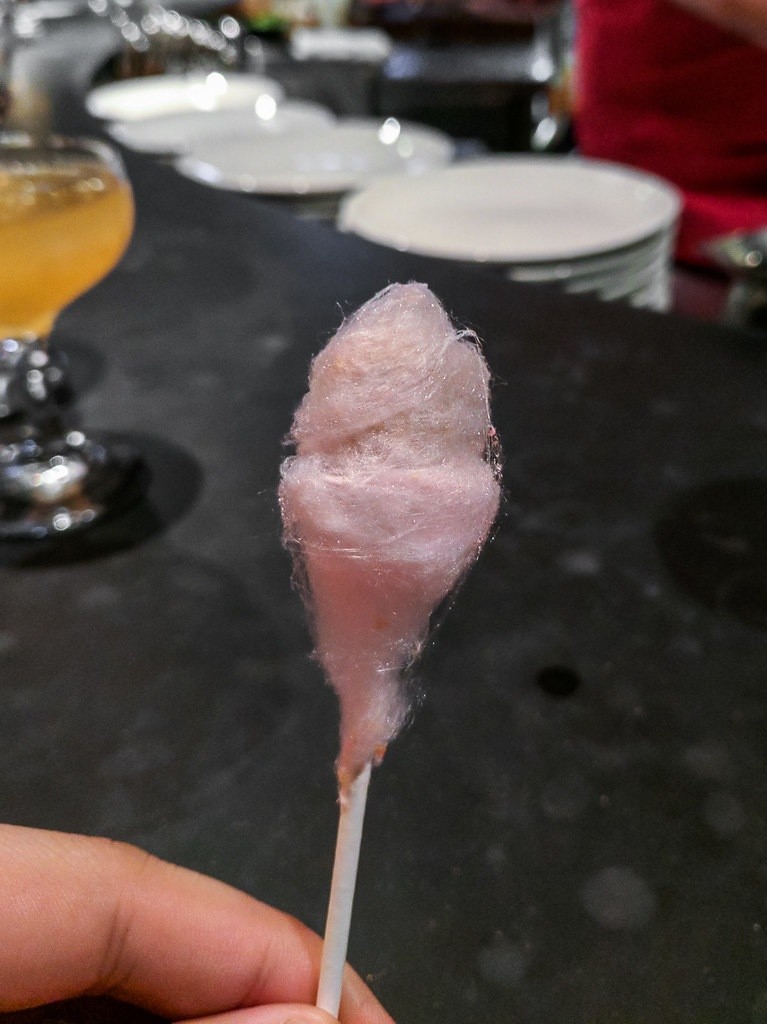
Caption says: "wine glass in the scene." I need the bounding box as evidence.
[0,132,136,540]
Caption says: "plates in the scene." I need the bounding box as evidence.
[83,69,679,315]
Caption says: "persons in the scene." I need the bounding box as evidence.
[0,825,397,1024]
[344,0,767,276]
